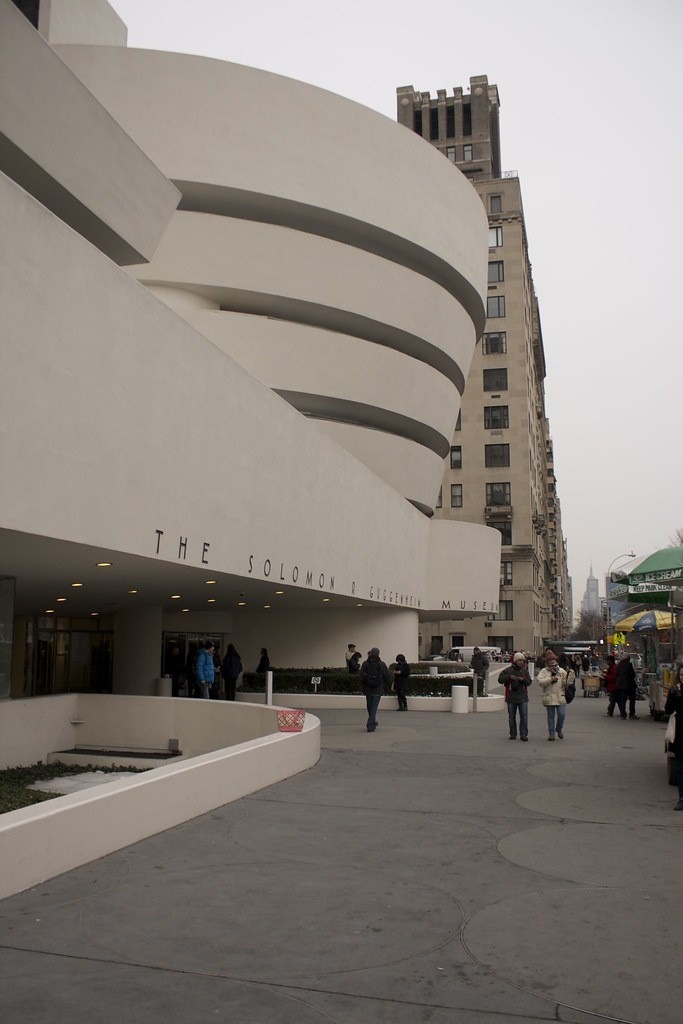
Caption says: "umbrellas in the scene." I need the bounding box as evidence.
[609,584,683,671]
[611,545,683,586]
[615,608,677,671]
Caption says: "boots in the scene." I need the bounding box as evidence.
[396,701,402,712]
[402,698,407,711]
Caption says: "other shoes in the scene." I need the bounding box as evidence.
[629,714,641,720]
[556,729,563,740]
[674,800,683,810]
[368,721,378,732]
[509,732,516,739]
[549,734,556,740]
[521,735,528,741]
[603,713,612,717]
[621,713,628,718]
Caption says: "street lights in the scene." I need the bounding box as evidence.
[607,553,636,658]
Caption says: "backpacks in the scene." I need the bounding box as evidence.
[364,658,381,687]
[192,651,206,675]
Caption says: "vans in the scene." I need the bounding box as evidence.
[433,646,502,664]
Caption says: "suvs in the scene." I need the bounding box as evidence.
[615,651,645,669]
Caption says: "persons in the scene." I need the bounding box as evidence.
[161,641,241,701]
[394,655,410,712]
[471,647,489,679]
[256,648,270,672]
[536,650,575,741]
[360,648,389,732]
[346,644,370,674]
[601,654,640,720]
[440,649,529,663]
[664,659,683,811]
[538,651,598,678]
[498,652,532,741]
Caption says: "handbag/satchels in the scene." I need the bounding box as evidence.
[664,709,676,742]
[565,671,575,703]
[602,679,607,688]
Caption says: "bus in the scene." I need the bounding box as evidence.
[654,642,678,672]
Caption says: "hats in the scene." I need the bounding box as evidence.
[545,650,555,662]
[513,652,526,663]
[206,641,214,650]
